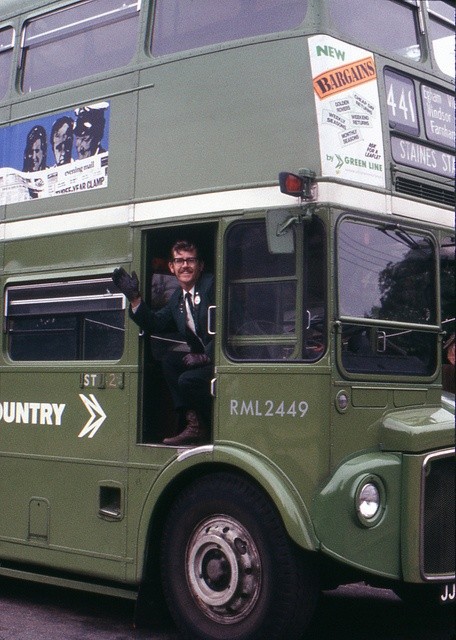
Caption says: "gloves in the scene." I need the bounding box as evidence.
[112,268,140,301]
[183,352,211,368]
[170,258,199,267]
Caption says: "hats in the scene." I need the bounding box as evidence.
[73,101,109,136]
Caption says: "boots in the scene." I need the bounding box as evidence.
[163,408,210,445]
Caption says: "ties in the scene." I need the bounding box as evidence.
[186,293,196,325]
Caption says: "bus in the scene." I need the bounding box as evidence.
[0,0,456,638]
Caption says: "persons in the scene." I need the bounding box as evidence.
[21,125,49,173]
[49,116,80,171]
[111,239,219,445]
[72,102,106,161]
